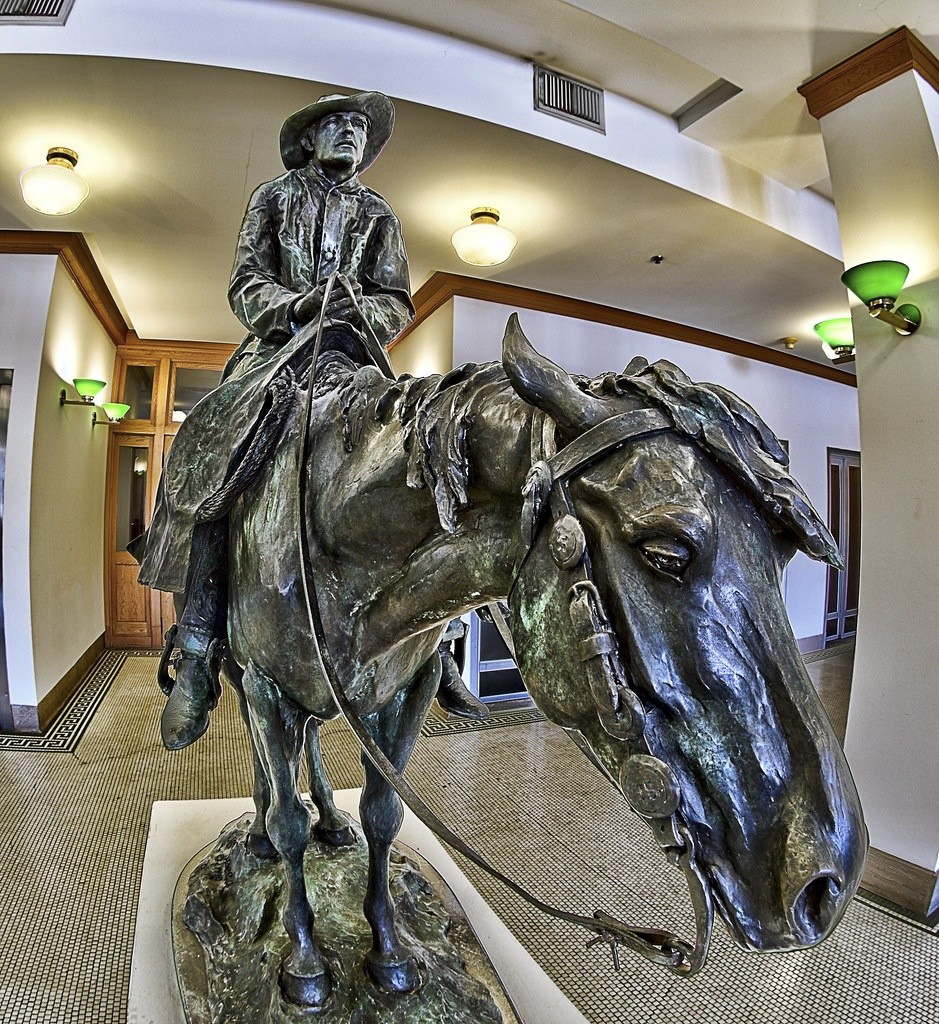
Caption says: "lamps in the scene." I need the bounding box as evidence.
[20,146,90,217]
[91,403,131,425]
[813,317,856,364]
[59,379,108,406]
[451,206,517,267]
[133,447,148,477]
[841,259,922,336]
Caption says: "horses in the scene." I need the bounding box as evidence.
[185,310,871,1007]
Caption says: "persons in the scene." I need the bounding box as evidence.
[126,92,490,751]
